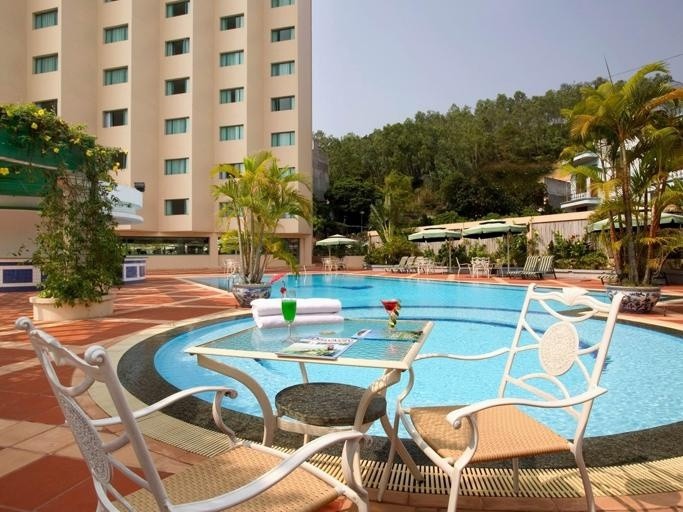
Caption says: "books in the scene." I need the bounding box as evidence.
[274,335,356,360]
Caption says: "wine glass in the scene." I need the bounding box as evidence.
[279,286,298,344]
[380,296,399,334]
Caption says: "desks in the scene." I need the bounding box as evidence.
[182,319,434,508]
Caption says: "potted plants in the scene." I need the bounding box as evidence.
[209,151,316,305]
[556,59,683,314]
[9,141,129,320]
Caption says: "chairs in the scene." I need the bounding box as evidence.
[394,283,619,512]
[456,255,556,281]
[14,315,373,512]
[323,256,348,270]
[385,256,447,274]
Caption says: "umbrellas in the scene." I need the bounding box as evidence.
[584,211,683,234]
[461,219,530,273]
[405,224,461,271]
[315,233,358,246]
